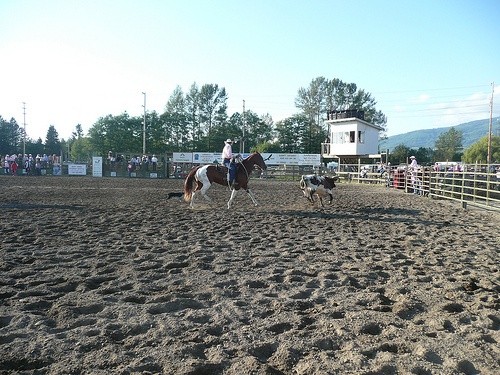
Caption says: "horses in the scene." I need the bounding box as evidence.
[183,150,267,210]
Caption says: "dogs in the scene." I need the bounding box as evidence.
[165,192,186,203]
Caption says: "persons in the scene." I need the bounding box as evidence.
[455,163,461,171]
[108,150,158,173]
[434,163,440,172]
[0,154,58,173]
[385,162,392,187]
[408,156,418,193]
[221,138,235,184]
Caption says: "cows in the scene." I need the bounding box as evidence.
[300,173,340,207]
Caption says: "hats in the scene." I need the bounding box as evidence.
[224,139,234,145]
[410,156,416,159]
